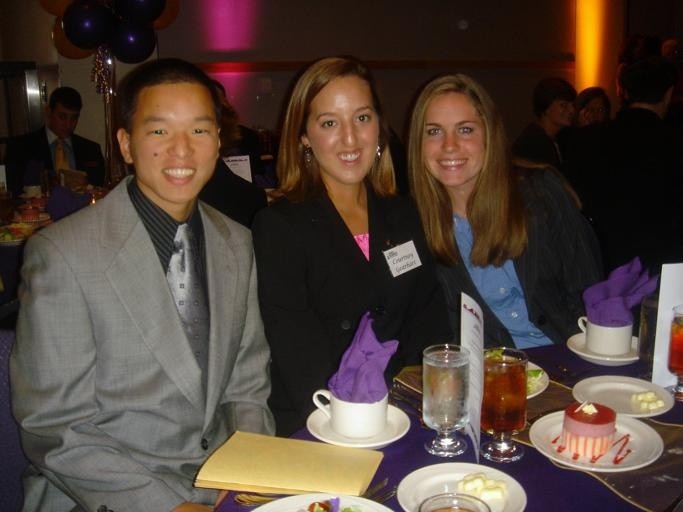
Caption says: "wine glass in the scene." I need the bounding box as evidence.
[479,347,528,464]
[664,305,682,401]
[419,343,469,460]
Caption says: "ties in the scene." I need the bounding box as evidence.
[55,140,69,169]
[166,223,208,371]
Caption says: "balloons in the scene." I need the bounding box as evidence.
[38,1,164,64]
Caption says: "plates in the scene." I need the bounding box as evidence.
[1,192,53,247]
[528,408,663,473]
[393,462,526,512]
[306,403,411,450]
[566,333,640,369]
[527,356,548,400]
[238,493,390,512]
[568,374,674,418]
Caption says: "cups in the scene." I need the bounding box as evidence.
[574,314,636,356]
[310,387,390,439]
[415,492,490,512]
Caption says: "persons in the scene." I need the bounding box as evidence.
[250,57,458,439]
[570,87,611,133]
[193,85,270,231]
[4,86,106,201]
[515,76,575,169]
[567,42,682,263]
[10,58,276,512]
[406,72,605,356]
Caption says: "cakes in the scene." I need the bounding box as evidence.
[563,403,617,458]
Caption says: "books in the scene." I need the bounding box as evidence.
[189,429,385,498]
